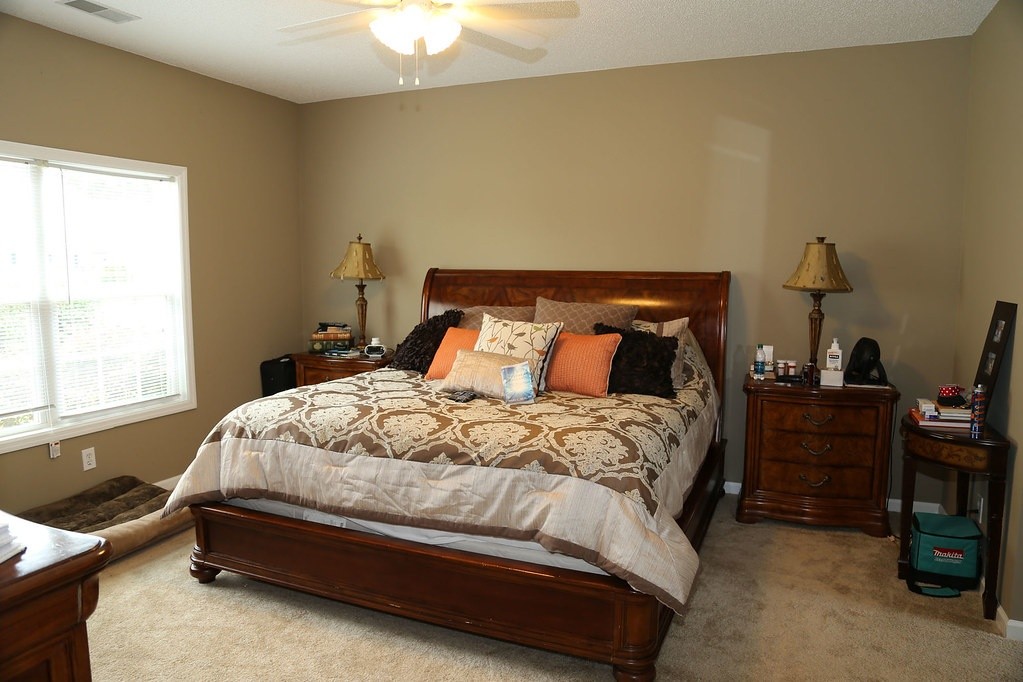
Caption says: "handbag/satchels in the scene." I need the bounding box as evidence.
[908,512,984,597]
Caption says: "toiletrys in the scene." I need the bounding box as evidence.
[826,338,842,370]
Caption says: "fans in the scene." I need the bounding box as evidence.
[277,0,580,85]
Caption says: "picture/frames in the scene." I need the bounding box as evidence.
[973,300,1018,419]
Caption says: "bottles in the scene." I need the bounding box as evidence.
[971,384,985,434]
[754,344,765,380]
[371,337,380,345]
[776,360,796,376]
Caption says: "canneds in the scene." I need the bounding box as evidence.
[776,360,797,376]
[802,363,815,386]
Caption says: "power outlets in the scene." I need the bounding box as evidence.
[82,446,96,471]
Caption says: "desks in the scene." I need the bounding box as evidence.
[0,508,114,682]
[896,412,1010,621]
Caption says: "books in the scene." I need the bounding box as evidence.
[931,400,972,419]
[0,523,27,565]
[311,331,352,340]
[308,337,355,354]
[907,407,971,427]
[501,361,535,405]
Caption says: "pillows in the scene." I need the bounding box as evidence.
[459,305,536,331]
[544,333,622,398]
[435,349,540,401]
[473,313,565,392]
[387,309,466,375]
[423,327,480,378]
[533,296,639,336]
[633,317,689,390]
[594,323,678,400]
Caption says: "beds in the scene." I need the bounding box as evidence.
[189,267,733,682]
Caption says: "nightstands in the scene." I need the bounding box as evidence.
[736,373,901,537]
[291,350,394,387]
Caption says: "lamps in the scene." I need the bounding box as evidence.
[368,0,463,85]
[329,233,386,353]
[782,236,854,384]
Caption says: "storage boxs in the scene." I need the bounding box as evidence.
[820,368,845,387]
[915,398,935,414]
